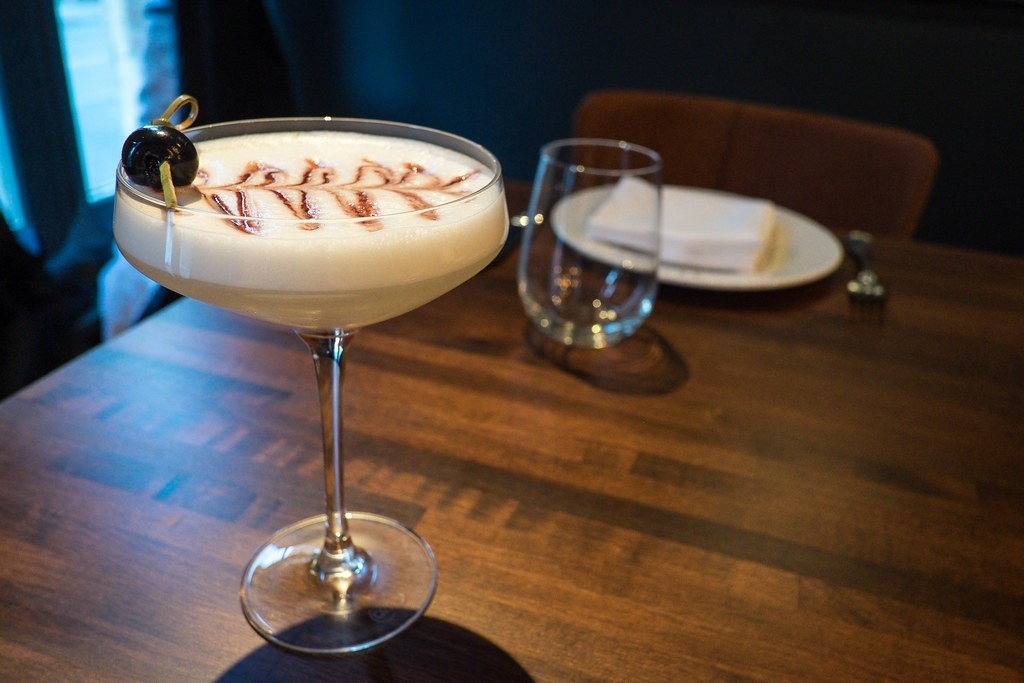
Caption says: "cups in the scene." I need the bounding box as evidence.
[519,138,661,351]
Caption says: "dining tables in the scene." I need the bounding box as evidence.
[0,181,1024,683]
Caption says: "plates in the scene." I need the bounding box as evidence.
[550,185,844,290]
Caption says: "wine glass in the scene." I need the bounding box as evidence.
[111,114,511,654]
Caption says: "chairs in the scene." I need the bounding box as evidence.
[573,86,944,243]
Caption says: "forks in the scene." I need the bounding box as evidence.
[844,231,888,318]
